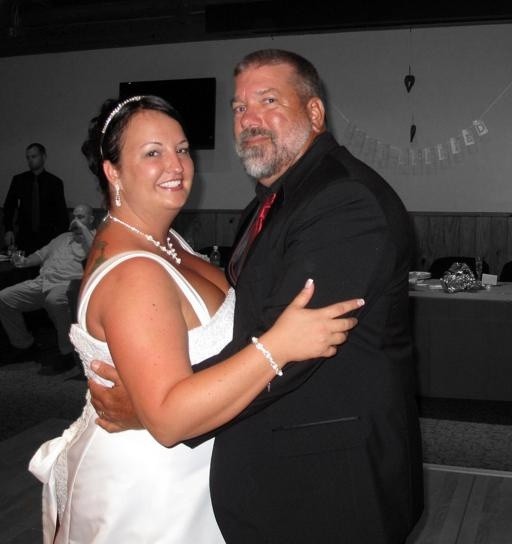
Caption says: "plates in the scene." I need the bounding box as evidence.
[409,270,432,279]
[429,284,442,289]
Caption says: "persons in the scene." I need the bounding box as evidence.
[1,143,69,256]
[28,96,367,541]
[83,48,421,543]
[0,205,98,371]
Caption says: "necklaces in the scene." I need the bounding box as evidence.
[101,212,183,266]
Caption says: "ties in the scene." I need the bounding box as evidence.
[30,175,42,233]
[228,190,282,285]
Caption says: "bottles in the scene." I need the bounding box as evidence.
[210,245,221,264]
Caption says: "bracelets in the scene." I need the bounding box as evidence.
[249,335,284,379]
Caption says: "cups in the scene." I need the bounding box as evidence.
[13,250,25,266]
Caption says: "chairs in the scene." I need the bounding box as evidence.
[497,259,512,282]
[427,253,478,282]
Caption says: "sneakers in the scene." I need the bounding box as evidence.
[62,351,84,380]
[0,346,27,362]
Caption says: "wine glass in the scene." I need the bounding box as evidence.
[475,254,482,281]
